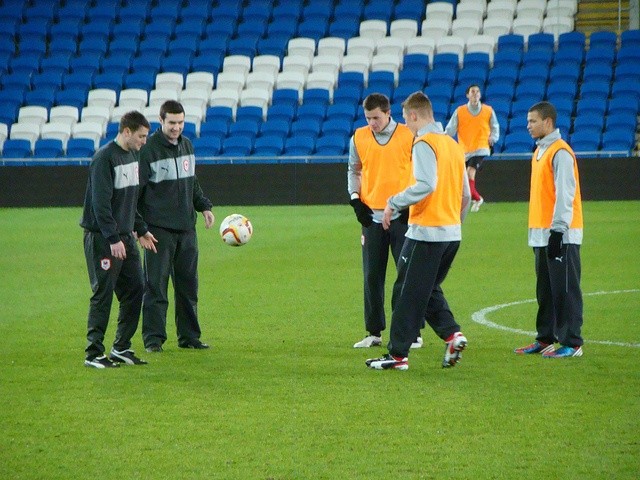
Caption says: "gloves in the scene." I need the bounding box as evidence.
[548,230,564,259]
[349,198,374,227]
[399,207,409,224]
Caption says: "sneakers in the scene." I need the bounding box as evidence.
[146,344,163,352]
[84,354,120,368]
[353,335,382,348]
[178,337,209,348]
[542,346,583,358]
[514,340,555,354]
[365,354,409,370]
[471,196,483,212]
[442,331,468,368]
[109,347,148,364]
[410,337,422,348]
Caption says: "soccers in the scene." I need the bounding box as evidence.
[220,213,253,247]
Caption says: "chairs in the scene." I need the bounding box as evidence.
[391,97,413,119]
[140,33,168,53]
[18,106,48,132]
[303,5,329,26]
[271,4,299,24]
[620,29,640,46]
[497,34,524,51]
[426,2,452,33]
[78,35,108,56]
[195,122,227,148]
[528,33,553,46]
[92,66,124,94]
[512,100,534,117]
[513,17,541,42]
[523,47,552,62]
[454,80,483,101]
[19,19,54,40]
[542,15,574,46]
[590,31,616,43]
[10,50,40,73]
[493,50,521,69]
[325,95,359,119]
[554,43,585,60]
[520,62,548,80]
[463,51,489,64]
[113,103,142,124]
[493,130,503,153]
[546,78,576,97]
[206,23,235,40]
[459,61,487,80]
[267,104,294,134]
[330,21,356,48]
[342,56,369,88]
[0,13,16,33]
[549,60,580,79]
[185,71,213,100]
[466,36,494,66]
[86,88,117,115]
[0,50,10,68]
[149,121,160,135]
[611,80,640,98]
[290,119,320,149]
[218,137,252,163]
[101,122,119,146]
[180,5,209,24]
[238,23,266,39]
[198,37,227,61]
[192,136,220,163]
[63,68,92,94]
[17,31,46,52]
[556,108,571,127]
[176,18,205,40]
[134,48,161,71]
[353,118,369,132]
[585,43,616,63]
[31,68,62,91]
[67,137,94,158]
[316,132,347,158]
[496,109,509,132]
[170,33,198,57]
[71,49,102,70]
[24,1,61,23]
[487,1,515,20]
[556,126,568,142]
[229,122,258,150]
[451,101,466,115]
[435,41,464,68]
[102,47,132,69]
[149,89,179,106]
[484,18,508,39]
[427,68,454,97]
[259,120,288,151]
[274,90,297,115]
[246,73,273,105]
[181,93,208,123]
[48,34,77,56]
[601,130,633,156]
[503,132,533,152]
[580,78,609,99]
[0,123,8,150]
[34,138,63,157]
[10,123,39,152]
[82,106,109,132]
[211,89,238,123]
[334,5,360,23]
[259,34,289,62]
[1,70,32,90]
[576,97,606,116]
[40,121,70,150]
[249,146,280,164]
[605,111,635,145]
[421,19,448,39]
[2,139,31,157]
[616,45,640,64]
[165,51,189,80]
[609,97,638,125]
[299,22,325,43]
[119,1,147,23]
[359,19,385,51]
[452,19,479,43]
[252,55,279,86]
[485,84,515,101]
[398,65,426,95]
[146,18,174,37]
[574,113,604,133]
[56,2,90,21]
[40,47,72,70]
[509,116,530,134]
[151,1,178,24]
[82,18,112,38]
[547,94,573,112]
[364,4,392,32]
[241,89,268,121]
[428,96,449,121]
[156,71,183,95]
[394,3,422,36]
[223,56,250,85]
[312,57,339,88]
[390,18,418,49]
[559,31,584,44]
[207,106,232,134]
[216,72,243,100]
[377,40,406,70]
[347,36,375,64]
[26,90,54,117]
[119,88,147,111]
[369,72,393,99]
[287,37,316,68]
[456,2,483,31]
[486,100,511,118]
[210,4,240,24]
[583,62,612,81]
[335,72,367,98]
[87,3,119,21]
[570,131,600,155]
[277,73,305,103]
[124,68,153,95]
[406,39,434,69]
[310,72,335,103]
[0,99,18,131]
[1,2,29,20]
[516,80,545,100]
[278,59,306,92]
[109,32,140,51]
[192,55,222,85]
[235,104,262,133]
[0,31,14,51]
[242,7,267,25]
[516,0,545,16]
[113,17,142,39]
[373,55,399,90]
[229,39,256,62]
[74,123,102,148]
[51,20,84,38]
[325,104,357,134]
[614,62,640,81]
[433,53,458,83]
[56,84,86,107]
[488,68,518,85]
[0,83,26,104]
[49,104,79,132]
[303,89,329,114]
[282,136,316,159]
[181,121,195,140]
[317,37,344,69]
[297,103,325,134]
[144,105,161,124]
[546,0,577,17]
[404,53,431,83]
[267,16,297,35]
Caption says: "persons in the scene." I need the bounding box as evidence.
[443,85,500,212]
[80,111,150,368]
[132,100,214,353]
[515,102,584,358]
[364,89,471,369]
[347,93,422,348]
